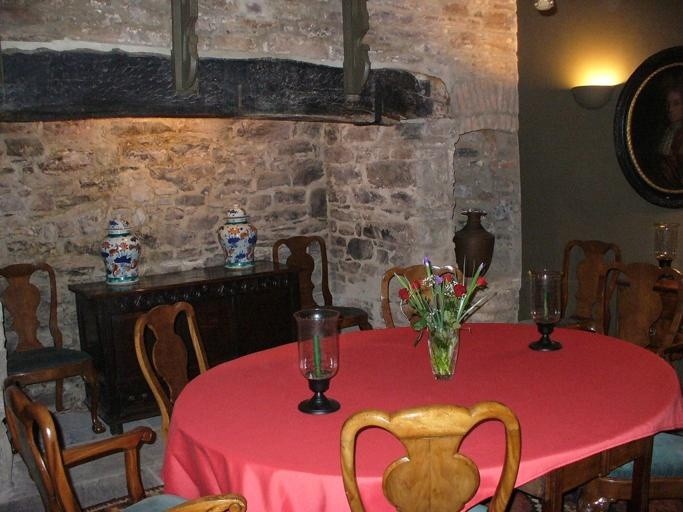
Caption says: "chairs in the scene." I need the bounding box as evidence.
[380,264,463,328]
[340,400,522,512]
[554,238,622,335]
[133,301,210,446]
[0,262,107,434]
[273,236,372,336]
[4,385,248,512]
[576,261,683,512]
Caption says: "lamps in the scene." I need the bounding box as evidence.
[572,85,611,108]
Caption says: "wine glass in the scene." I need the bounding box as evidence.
[291,308,341,415]
[528,267,565,353]
[652,221,679,280]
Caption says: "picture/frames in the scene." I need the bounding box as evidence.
[613,45,683,210]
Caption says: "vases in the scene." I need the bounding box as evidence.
[428,326,460,380]
[453,207,495,278]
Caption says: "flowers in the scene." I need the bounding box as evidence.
[394,255,491,376]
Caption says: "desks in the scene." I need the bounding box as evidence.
[67,260,304,434]
[615,280,683,346]
